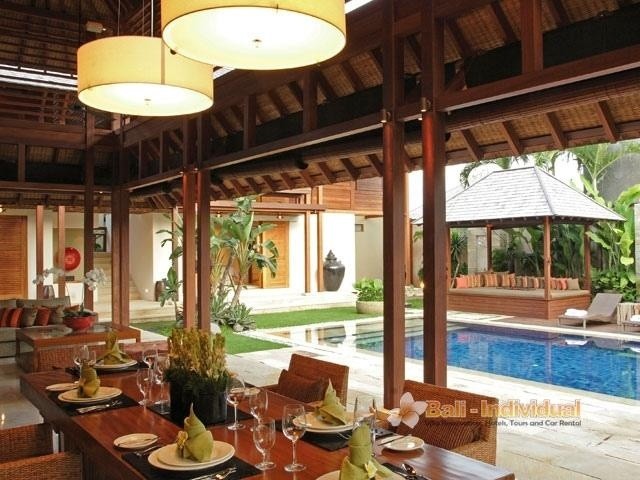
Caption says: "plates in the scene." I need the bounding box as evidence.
[316,466,405,480]
[92,357,137,371]
[59,386,123,404]
[113,433,159,451]
[147,441,236,472]
[379,435,425,452]
[244,387,261,398]
[45,382,74,391]
[292,412,356,434]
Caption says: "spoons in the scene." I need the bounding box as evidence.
[402,463,432,480]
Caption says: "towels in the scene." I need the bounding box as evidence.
[102,330,133,366]
[338,421,390,480]
[74,358,102,400]
[174,400,216,463]
[310,376,349,424]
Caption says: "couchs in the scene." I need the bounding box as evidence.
[0,297,76,360]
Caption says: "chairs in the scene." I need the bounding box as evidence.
[371,379,502,467]
[0,418,88,480]
[254,351,351,421]
[558,291,623,332]
[617,300,640,332]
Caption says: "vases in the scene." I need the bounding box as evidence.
[169,380,227,426]
[43,287,56,299]
[63,316,95,330]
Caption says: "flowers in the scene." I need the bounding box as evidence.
[366,391,428,433]
[32,264,65,287]
[160,323,231,397]
[63,266,108,316]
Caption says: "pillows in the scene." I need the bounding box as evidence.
[275,366,330,404]
[395,411,483,450]
[44,302,66,325]
[32,305,50,326]
[19,307,37,327]
[453,268,582,294]
[1,306,21,327]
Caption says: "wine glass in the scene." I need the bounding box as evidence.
[223,374,246,431]
[252,417,277,472]
[279,404,307,473]
[71,344,90,378]
[249,388,269,433]
[134,343,171,409]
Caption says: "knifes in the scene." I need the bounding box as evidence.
[189,467,237,480]
[380,462,414,480]
[377,433,409,446]
[134,444,162,456]
[75,399,122,414]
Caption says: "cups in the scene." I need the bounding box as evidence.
[352,395,376,432]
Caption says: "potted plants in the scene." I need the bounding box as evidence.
[351,276,385,314]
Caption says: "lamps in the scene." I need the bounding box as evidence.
[75,0,216,120]
[158,0,349,75]
